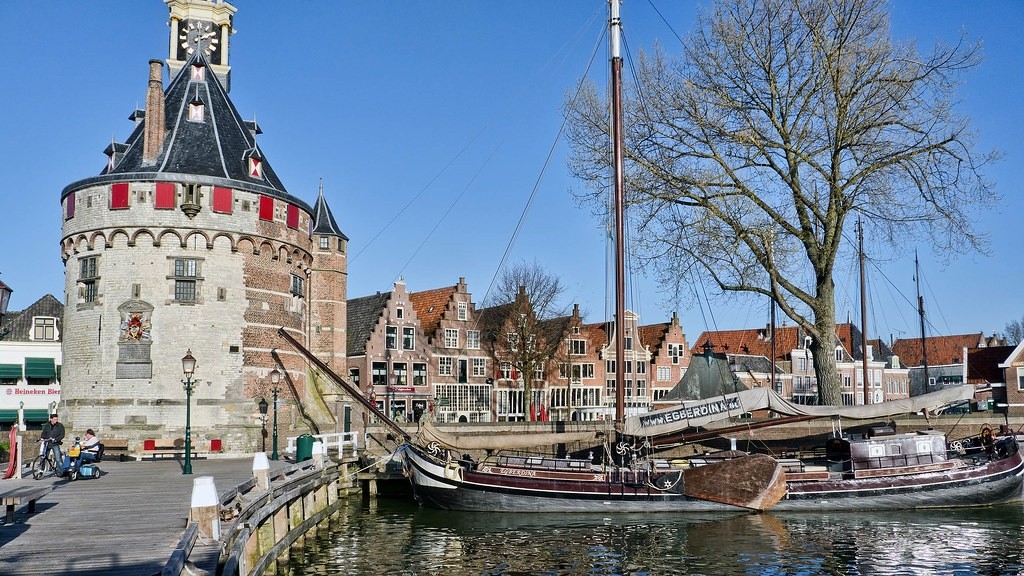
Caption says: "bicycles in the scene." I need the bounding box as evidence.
[32,437,69,480]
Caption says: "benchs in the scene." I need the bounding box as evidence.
[500,456,591,472]
[100,440,128,462]
[142,439,206,461]
[0,486,52,524]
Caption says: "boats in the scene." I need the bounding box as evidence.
[278,0,1021,517]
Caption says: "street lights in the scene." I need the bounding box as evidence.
[179,348,198,475]
[258,397,269,454]
[270,363,279,463]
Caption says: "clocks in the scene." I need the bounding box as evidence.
[178,19,221,66]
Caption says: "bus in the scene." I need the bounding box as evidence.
[0,338,65,429]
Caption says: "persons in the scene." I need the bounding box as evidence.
[37,414,65,477]
[62,429,101,472]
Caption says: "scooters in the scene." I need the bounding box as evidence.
[61,437,106,481]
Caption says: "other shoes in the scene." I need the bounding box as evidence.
[60,472,64,478]
[63,470,70,476]
[35,469,43,477]
